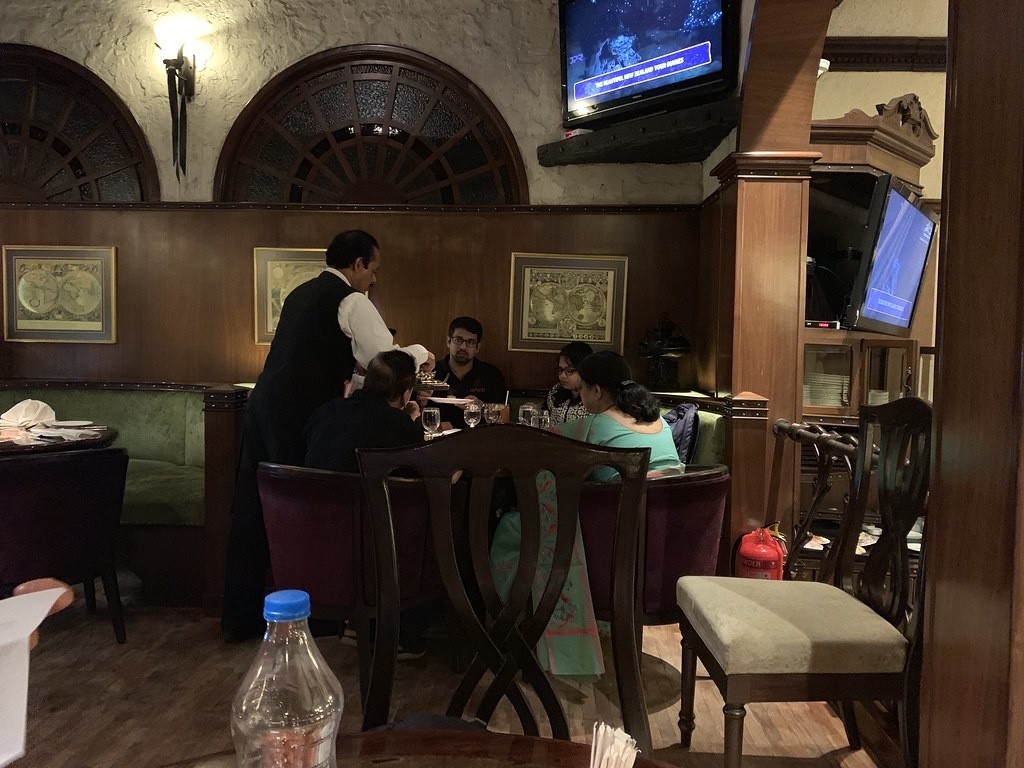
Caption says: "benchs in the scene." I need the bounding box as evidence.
[512,392,767,578]
[0,380,248,618]
[254,463,732,677]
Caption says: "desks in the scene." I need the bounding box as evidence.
[0,425,118,459]
[138,730,675,768]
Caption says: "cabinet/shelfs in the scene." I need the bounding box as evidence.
[805,328,932,634]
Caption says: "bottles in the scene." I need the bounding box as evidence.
[230,591,344,768]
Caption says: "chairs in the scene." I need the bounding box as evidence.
[355,423,654,750]
[0,445,130,652]
[675,395,932,768]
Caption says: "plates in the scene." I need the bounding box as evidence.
[855,546,867,555]
[858,535,879,547]
[907,530,922,539]
[803,541,823,550]
[812,535,830,544]
[51,421,93,429]
[870,390,890,405]
[802,385,811,405]
[426,398,475,404]
[862,523,875,530]
[869,528,883,535]
[907,543,921,552]
[804,373,850,407]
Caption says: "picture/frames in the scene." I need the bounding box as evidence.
[3,245,118,342]
[507,252,628,358]
[254,247,369,346]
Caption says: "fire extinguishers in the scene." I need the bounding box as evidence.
[731,520,789,580]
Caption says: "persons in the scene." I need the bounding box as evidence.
[417,318,513,529]
[489,351,687,676]
[525,341,593,432]
[221,230,435,636]
[344,328,396,398]
[303,350,428,661]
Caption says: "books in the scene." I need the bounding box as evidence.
[803,372,850,406]
[868,389,903,406]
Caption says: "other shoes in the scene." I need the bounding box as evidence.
[220,622,263,642]
[395,638,427,661]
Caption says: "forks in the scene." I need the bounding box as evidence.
[26,423,37,430]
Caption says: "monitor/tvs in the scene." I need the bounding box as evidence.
[842,173,936,337]
[558,0,744,133]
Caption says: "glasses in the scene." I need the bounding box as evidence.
[554,366,578,375]
[450,336,479,348]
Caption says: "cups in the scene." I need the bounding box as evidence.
[531,410,549,430]
[484,403,503,424]
[518,405,532,425]
[464,404,482,428]
[503,404,509,423]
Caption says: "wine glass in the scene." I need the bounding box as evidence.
[422,408,440,440]
